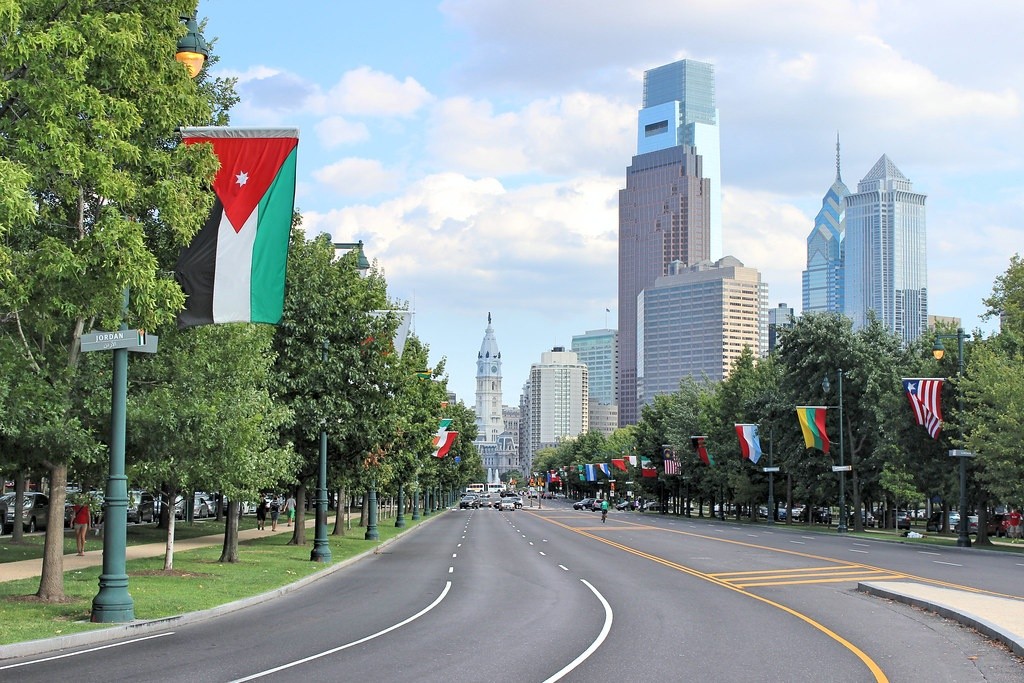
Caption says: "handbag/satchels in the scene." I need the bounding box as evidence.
[71,518,75,529]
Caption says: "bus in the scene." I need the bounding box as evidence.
[485,482,507,493]
[466,483,484,493]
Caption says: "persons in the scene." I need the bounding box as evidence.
[1009,507,1023,544]
[600,499,609,520]
[255,493,296,531]
[70,504,91,556]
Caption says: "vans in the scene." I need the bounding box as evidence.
[926,510,960,532]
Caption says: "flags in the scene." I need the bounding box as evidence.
[360,310,411,366]
[692,436,718,464]
[156,125,299,331]
[432,401,458,457]
[533,454,657,487]
[796,406,830,452]
[736,424,763,463]
[902,379,943,439]
[662,444,682,475]
[415,370,433,380]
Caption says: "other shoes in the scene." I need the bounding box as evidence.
[258,524,260,530]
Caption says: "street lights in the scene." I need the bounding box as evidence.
[822,367,848,532]
[932,328,971,547]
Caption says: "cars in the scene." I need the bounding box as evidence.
[458,490,568,509]
[953,516,979,534]
[635,496,667,511]
[713,503,832,524]
[572,498,595,510]
[498,498,515,511]
[849,511,875,527]
[591,499,604,512]
[66,488,286,528]
[986,512,1024,537]
[615,501,635,511]
[0,499,9,537]
[878,508,911,530]
[0,491,51,533]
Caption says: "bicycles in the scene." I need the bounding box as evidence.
[601,509,607,523]
